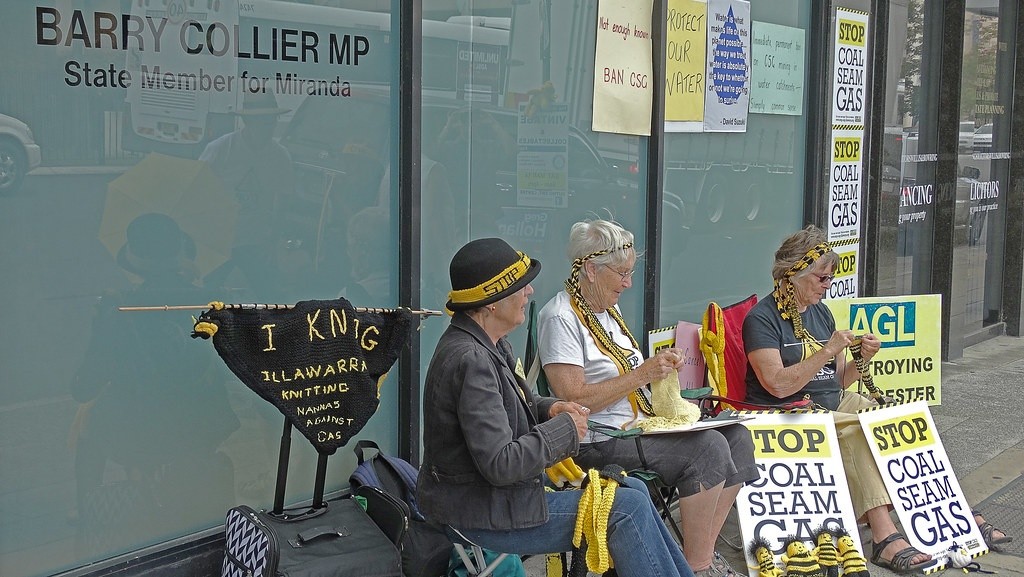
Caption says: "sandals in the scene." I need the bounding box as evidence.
[870,532,937,574]
[972,509,1013,543]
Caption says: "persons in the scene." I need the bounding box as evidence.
[414,219,760,577]
[741,224,1013,572]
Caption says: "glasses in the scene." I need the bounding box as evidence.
[604,265,636,282]
[812,272,835,283]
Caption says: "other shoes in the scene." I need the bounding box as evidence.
[694,551,749,577]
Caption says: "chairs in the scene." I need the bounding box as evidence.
[701,293,898,552]
[525,301,715,549]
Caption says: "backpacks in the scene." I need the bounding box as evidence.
[348,439,453,577]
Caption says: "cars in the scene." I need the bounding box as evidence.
[959,122,975,153]
[869,126,992,249]
[265,78,683,293]
[0,113,43,194]
[972,123,993,150]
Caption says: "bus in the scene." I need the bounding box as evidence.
[120,0,514,155]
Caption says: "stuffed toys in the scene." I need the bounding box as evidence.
[745,526,870,577]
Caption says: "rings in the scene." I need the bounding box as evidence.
[582,406,591,413]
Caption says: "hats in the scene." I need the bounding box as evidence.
[446,238,541,311]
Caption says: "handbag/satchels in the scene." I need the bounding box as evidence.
[221,416,403,577]
[449,543,526,577]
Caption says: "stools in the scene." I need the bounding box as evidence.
[441,523,534,577]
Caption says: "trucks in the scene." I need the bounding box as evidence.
[500,0,807,232]
